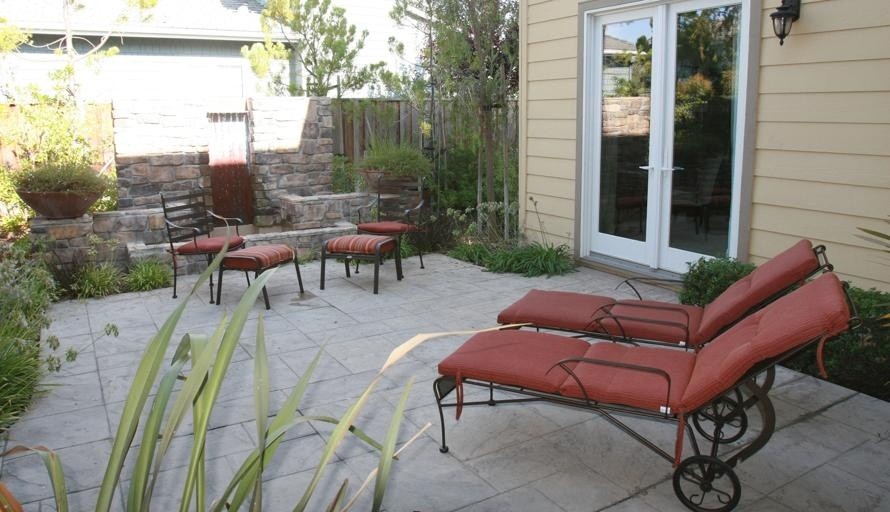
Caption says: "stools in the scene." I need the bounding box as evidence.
[214,243,303,309]
[319,234,402,295]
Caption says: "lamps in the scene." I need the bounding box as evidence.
[770,0,801,46]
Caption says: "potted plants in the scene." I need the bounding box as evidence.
[6,160,110,220]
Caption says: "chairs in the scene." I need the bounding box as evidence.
[431,272,850,512]
[160,189,250,304]
[354,175,424,279]
[493,237,834,407]
